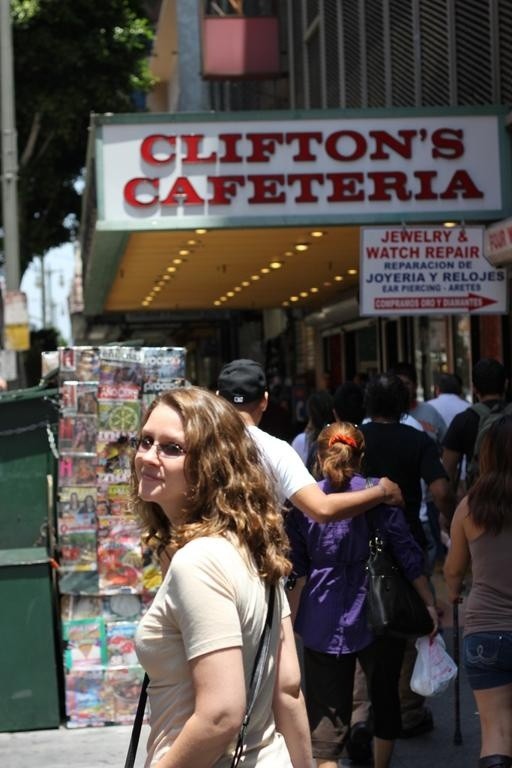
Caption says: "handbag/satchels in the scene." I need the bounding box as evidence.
[366,476,433,634]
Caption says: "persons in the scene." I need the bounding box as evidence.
[281,416,440,768]
[132,385,318,768]
[351,369,461,742]
[217,358,406,520]
[441,415,512,768]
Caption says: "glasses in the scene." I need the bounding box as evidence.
[132,437,187,458]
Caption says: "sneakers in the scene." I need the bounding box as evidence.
[348,722,374,768]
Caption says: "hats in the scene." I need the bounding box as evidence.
[218,359,267,404]
